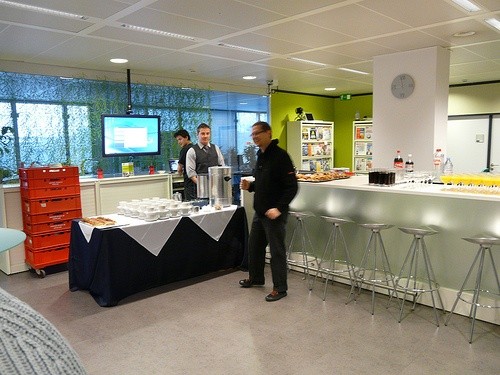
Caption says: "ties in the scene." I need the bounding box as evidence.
[203,145,209,151]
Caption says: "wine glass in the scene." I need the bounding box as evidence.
[404,170,436,190]
[440,174,500,195]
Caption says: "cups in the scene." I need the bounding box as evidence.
[368,167,404,188]
[117,192,193,220]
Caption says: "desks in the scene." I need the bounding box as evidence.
[69,204,250,308]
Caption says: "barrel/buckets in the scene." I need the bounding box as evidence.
[208,163,232,207]
[195,174,209,199]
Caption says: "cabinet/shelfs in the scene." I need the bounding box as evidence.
[287,120,334,171]
[352,121,373,174]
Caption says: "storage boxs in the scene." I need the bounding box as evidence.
[19,165,82,269]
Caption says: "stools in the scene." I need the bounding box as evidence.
[312,215,359,301]
[286,210,325,290]
[386,228,446,327]
[444,238,500,344]
[345,224,400,314]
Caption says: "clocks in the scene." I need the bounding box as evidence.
[391,74,415,99]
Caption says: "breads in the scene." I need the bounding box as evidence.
[296,172,349,181]
[81,217,115,226]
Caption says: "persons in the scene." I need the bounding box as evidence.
[174,129,198,202]
[186,123,226,184]
[239,121,299,302]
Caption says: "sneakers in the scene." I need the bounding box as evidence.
[265,291,287,301]
[239,279,265,286]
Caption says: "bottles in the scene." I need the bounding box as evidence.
[149,166,154,174]
[442,157,453,174]
[405,154,414,173]
[97,169,103,178]
[433,149,444,180]
[393,150,404,169]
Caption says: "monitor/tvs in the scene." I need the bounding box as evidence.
[101,113,161,157]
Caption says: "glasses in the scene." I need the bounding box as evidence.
[251,130,266,137]
